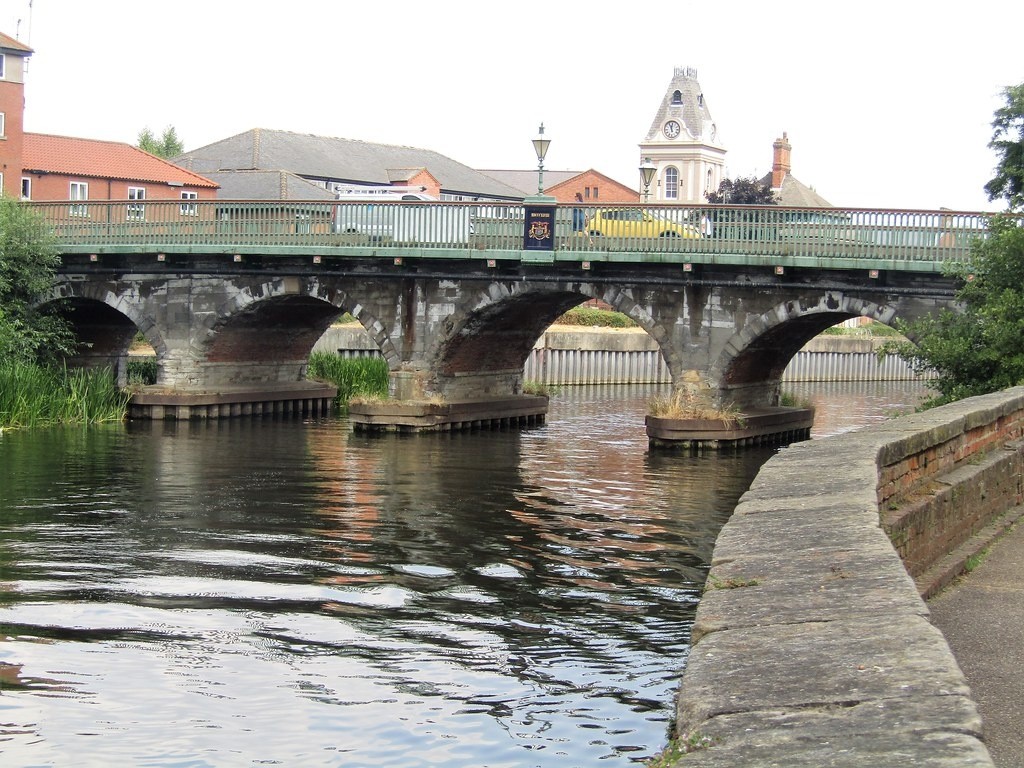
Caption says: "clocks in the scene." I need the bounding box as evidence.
[708,123,717,143]
[664,120,681,139]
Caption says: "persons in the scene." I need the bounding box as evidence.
[561,193,595,246]
[699,212,711,238]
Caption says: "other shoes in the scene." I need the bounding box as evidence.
[562,244,569,247]
[590,242,594,247]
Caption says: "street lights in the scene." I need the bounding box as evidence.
[528,120,551,198]
[639,156,659,214]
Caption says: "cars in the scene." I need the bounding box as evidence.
[576,208,704,241]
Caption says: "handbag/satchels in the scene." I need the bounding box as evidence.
[585,214,589,227]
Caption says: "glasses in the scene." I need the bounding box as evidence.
[575,196,577,197]
